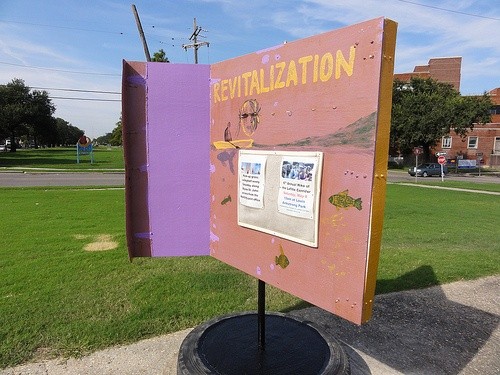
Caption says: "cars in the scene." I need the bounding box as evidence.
[408,162,448,177]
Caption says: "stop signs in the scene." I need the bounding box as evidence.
[438,156,445,164]
